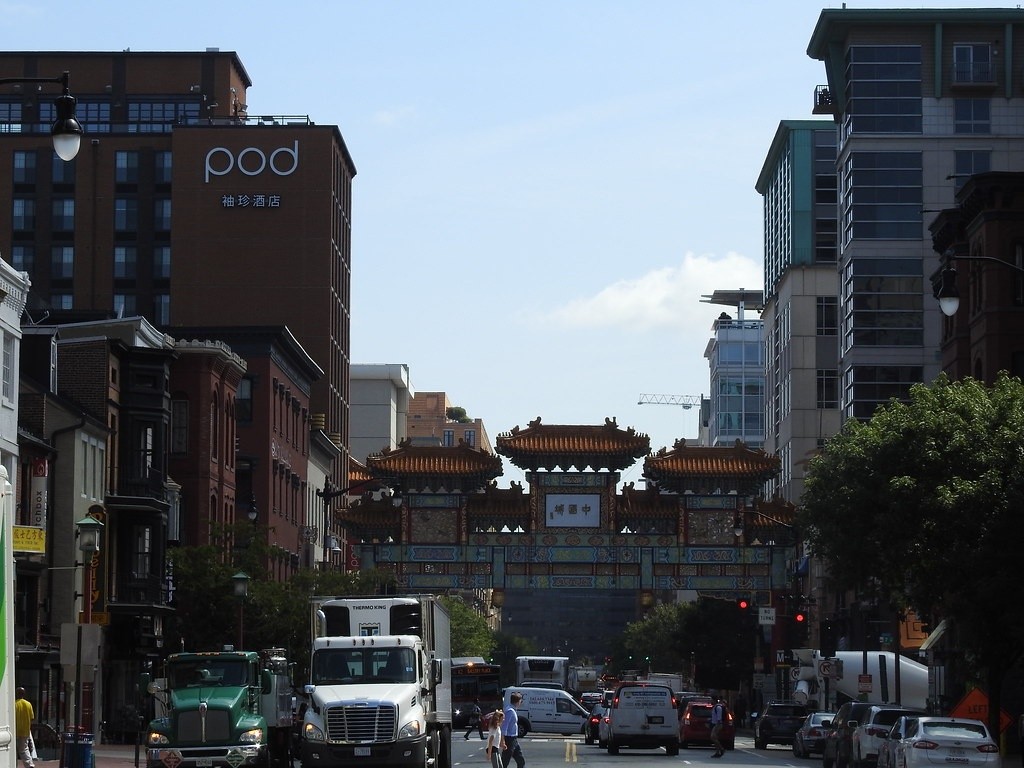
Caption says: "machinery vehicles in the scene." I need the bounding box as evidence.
[793,649,929,712]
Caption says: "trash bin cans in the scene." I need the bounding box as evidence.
[805,700,818,715]
[60,731,95,768]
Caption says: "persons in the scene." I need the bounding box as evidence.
[710,696,725,758]
[464,696,487,740]
[15,687,35,768]
[500,691,526,768]
[485,711,507,768]
[733,694,748,728]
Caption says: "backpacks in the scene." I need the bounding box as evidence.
[715,705,728,726]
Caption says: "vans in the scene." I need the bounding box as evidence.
[500,685,591,739]
[599,682,685,757]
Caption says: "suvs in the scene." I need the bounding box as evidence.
[847,704,933,768]
[751,697,811,750]
[820,700,901,768]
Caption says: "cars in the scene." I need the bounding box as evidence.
[513,655,737,751]
[792,712,837,759]
[875,714,1004,768]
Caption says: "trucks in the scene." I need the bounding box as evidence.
[137,642,301,768]
[294,593,455,768]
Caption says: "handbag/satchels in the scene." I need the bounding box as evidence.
[470,710,479,719]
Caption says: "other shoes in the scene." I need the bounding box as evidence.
[711,753,719,758]
[481,738,487,740]
[720,749,726,757]
[464,736,469,740]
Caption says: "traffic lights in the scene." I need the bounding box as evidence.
[794,610,809,643]
[736,596,752,629]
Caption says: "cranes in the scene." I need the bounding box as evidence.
[637,392,711,410]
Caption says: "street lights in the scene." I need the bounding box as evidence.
[314,473,405,585]
[732,508,817,648]
[244,457,261,544]
[76,513,103,736]
[232,570,250,651]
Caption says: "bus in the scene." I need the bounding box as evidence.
[450,656,504,730]
